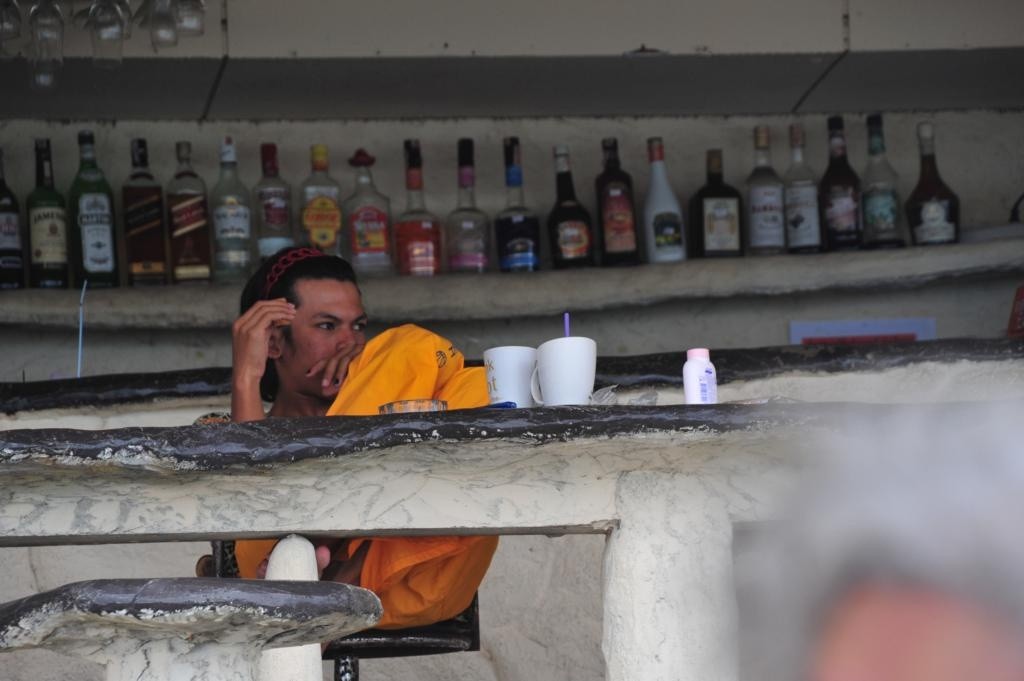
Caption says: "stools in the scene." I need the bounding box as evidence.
[0,575,383,681]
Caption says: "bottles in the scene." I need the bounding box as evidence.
[742,123,823,257]
[818,112,906,252]
[211,134,394,287]
[0,145,25,291]
[645,137,687,265]
[122,138,211,288]
[691,149,742,258]
[904,121,961,247]
[392,136,542,280]
[547,136,641,272]
[27,129,119,290]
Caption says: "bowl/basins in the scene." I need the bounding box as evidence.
[378,400,448,415]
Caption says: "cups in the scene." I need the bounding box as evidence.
[0,0,207,74]
[530,336,597,407]
[483,346,537,408]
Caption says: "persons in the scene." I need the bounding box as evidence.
[190,245,500,631]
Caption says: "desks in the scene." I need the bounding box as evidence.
[0,402,1024,681]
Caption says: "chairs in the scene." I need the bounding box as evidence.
[208,534,482,681]
